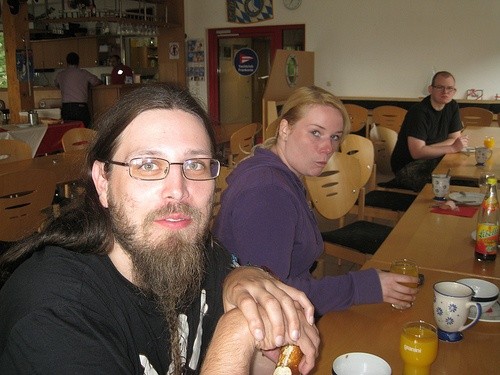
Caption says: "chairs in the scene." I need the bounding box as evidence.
[0,104,500,275]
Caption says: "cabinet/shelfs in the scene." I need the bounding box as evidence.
[30,36,98,69]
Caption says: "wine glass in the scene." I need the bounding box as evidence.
[118,23,160,36]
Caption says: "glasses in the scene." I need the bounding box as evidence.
[96,157,224,181]
[432,85,456,95]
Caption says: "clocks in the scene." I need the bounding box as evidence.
[283,0,301,10]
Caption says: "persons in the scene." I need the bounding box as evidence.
[390,71,469,199]
[109,54,133,85]
[0,78,321,375]
[51,52,100,129]
[205,85,420,336]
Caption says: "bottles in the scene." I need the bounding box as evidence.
[473,177,500,263]
[431,281,482,343]
[91,0,96,18]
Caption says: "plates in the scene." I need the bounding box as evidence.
[471,228,500,248]
[449,191,484,206]
[468,299,500,322]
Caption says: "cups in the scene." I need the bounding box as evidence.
[482,135,495,169]
[457,278,499,314]
[29,113,38,125]
[474,146,494,168]
[430,173,451,203]
[400,319,438,375]
[332,352,392,375]
[390,258,419,310]
[479,172,496,194]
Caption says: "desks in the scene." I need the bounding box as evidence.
[271,125,500,375]
[0,119,84,157]
